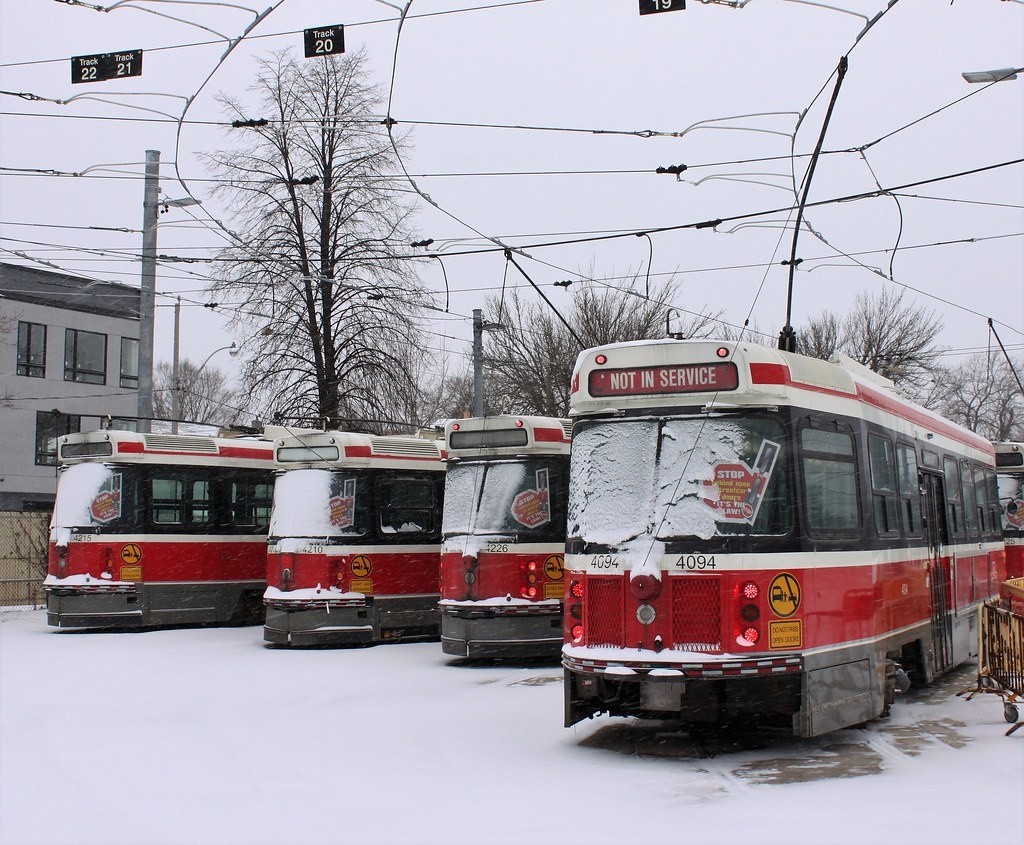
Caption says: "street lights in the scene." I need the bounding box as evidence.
[135,150,202,436]
[472,308,502,417]
[178,342,239,420]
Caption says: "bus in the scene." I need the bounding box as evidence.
[261,425,450,648]
[559,339,1007,743]
[42,431,278,629]
[439,413,572,663]
[989,440,1023,579]
